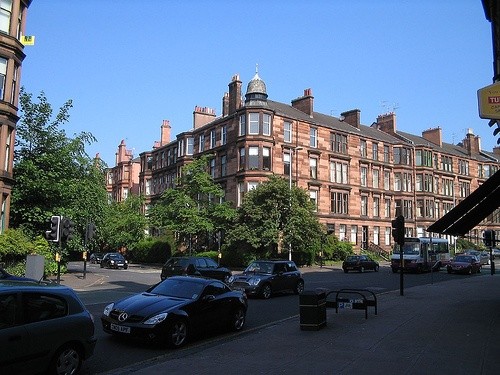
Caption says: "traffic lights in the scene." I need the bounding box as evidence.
[48,216,60,242]
[87,225,97,241]
[391,216,404,245]
[483,230,496,248]
[67,222,76,241]
[60,218,70,242]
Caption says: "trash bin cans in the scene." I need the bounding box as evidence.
[298,288,328,330]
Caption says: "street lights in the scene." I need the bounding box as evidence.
[288,146,303,260]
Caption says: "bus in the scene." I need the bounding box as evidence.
[390,237,450,273]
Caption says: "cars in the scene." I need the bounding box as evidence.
[101,276,249,348]
[342,255,379,272]
[483,249,496,259]
[468,252,491,266]
[90,253,105,264]
[101,253,128,270]
[229,260,304,299]
[161,258,233,284]
[0,280,97,375]
[447,255,482,275]
